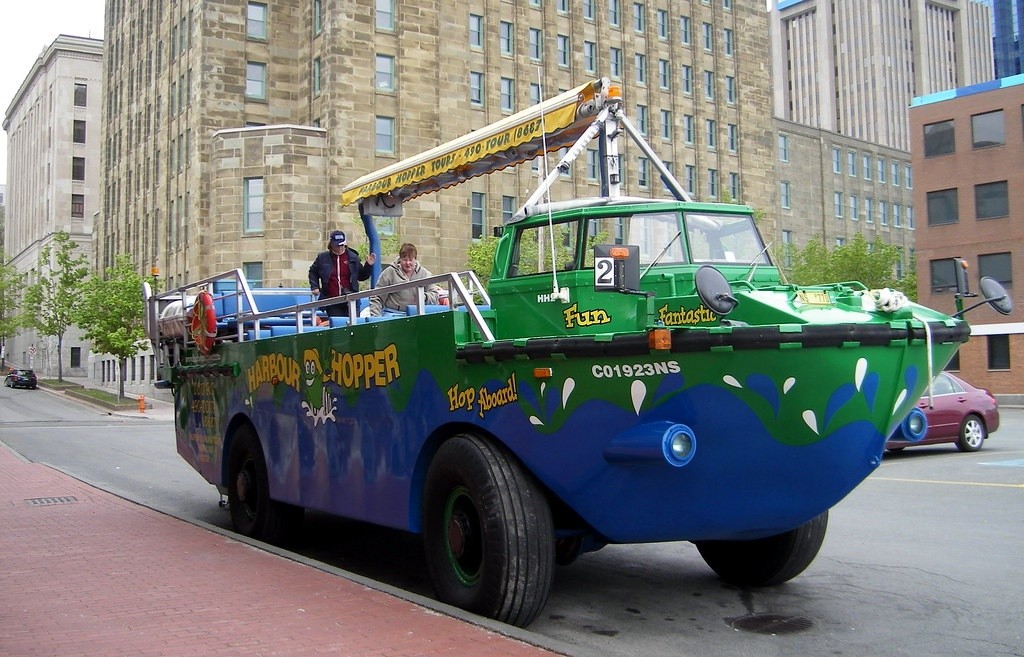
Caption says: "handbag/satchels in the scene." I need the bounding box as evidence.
[342,287,362,308]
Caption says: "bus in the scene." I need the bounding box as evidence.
[149,77,1019,628]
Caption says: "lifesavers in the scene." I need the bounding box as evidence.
[190,289,217,355]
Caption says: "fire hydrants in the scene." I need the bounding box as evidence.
[136,394,146,413]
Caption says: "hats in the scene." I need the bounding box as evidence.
[330,230,348,246]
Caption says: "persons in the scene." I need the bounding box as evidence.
[308,230,376,317]
[369,243,450,317]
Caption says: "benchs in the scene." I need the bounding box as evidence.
[212,288,491,341]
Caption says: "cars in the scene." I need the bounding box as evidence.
[876,367,1002,457]
[4,369,37,390]
